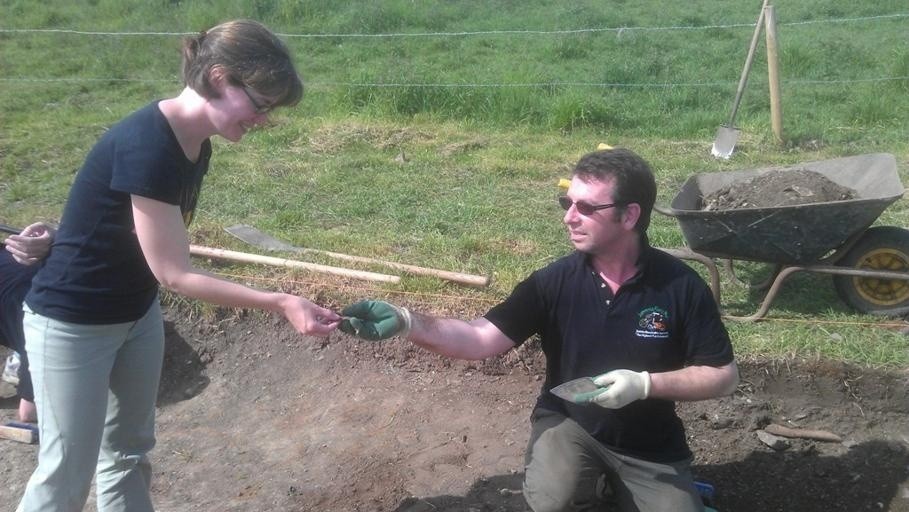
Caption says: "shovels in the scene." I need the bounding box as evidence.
[710,0,769,160]
[223,222,490,301]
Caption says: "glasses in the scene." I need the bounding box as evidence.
[242,87,271,116]
[558,195,617,216]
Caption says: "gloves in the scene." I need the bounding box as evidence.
[339,299,416,343]
[574,369,651,410]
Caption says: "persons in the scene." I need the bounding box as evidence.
[336,148,743,512]
[4,18,342,512]
[0,242,49,425]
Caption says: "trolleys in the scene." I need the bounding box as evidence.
[558,142,909,322]
